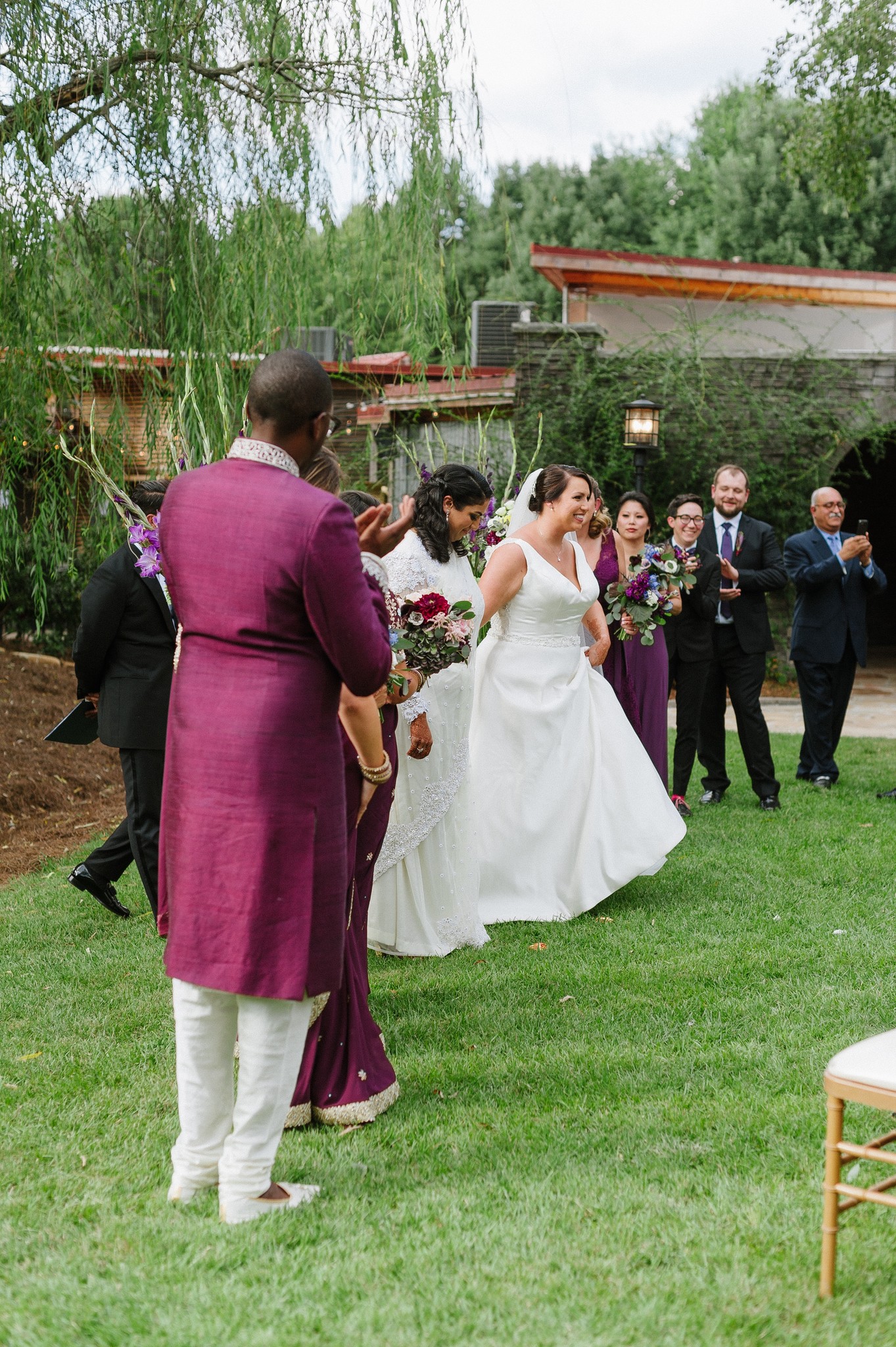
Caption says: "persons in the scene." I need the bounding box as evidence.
[607,492,682,798]
[651,492,721,818]
[43,480,178,943]
[157,350,416,1217]
[574,475,641,743]
[477,464,686,928]
[339,489,433,998]
[697,464,781,814]
[364,463,496,960]
[277,446,402,1130]
[783,486,887,791]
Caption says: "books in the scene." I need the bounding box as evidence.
[44,699,99,745]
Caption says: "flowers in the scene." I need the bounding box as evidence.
[603,568,680,645]
[393,591,475,685]
[627,539,703,595]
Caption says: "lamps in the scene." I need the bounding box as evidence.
[620,393,666,451]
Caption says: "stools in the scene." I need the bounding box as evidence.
[816,1027,896,1304]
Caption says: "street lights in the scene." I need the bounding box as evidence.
[624,393,662,493]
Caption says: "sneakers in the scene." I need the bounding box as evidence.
[672,798,693,817]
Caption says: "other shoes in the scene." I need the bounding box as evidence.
[224,1180,325,1225]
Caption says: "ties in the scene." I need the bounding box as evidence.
[719,522,733,619]
[828,535,841,557]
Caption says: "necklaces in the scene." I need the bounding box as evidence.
[535,520,563,562]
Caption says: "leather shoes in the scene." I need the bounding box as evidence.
[698,789,724,805]
[813,774,832,791]
[876,786,896,799]
[68,862,132,919]
[760,794,781,813]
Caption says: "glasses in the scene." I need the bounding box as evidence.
[815,501,847,512]
[677,514,705,526]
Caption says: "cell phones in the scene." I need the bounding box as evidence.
[857,519,869,536]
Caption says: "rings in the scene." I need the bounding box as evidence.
[416,748,424,753]
[633,626,637,631]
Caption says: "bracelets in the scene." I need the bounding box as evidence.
[859,557,871,568]
[410,666,427,691]
[354,750,393,783]
